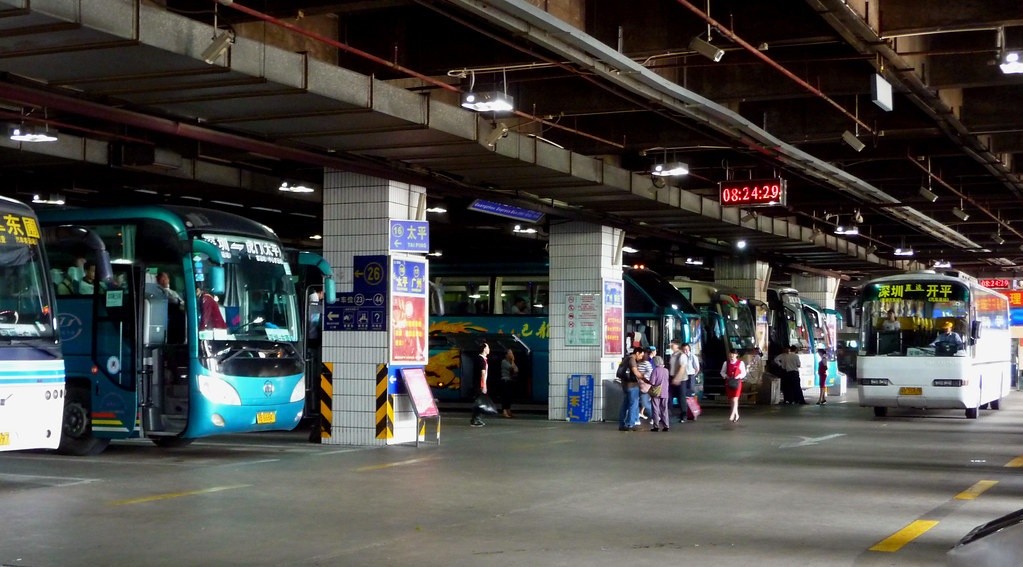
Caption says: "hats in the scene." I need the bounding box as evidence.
[942,322,953,328]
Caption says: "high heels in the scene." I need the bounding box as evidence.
[639,413,648,419]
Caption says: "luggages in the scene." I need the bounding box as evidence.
[687,396,702,421]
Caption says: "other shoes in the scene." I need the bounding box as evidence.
[619,426,628,431]
[470,420,486,427]
[651,428,660,431]
[662,427,669,431]
[680,419,686,423]
[627,426,643,432]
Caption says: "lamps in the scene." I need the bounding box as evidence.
[650,149,688,177]
[460,68,513,111]
[834,216,858,235]
[893,235,914,256]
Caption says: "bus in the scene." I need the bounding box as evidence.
[0,196,67,453]
[288,254,725,420]
[667,277,845,401]
[845,268,1013,418]
[0,203,339,454]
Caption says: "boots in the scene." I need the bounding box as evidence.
[502,409,511,418]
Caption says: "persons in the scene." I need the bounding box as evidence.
[618,346,669,432]
[929,321,962,350]
[512,297,531,314]
[720,349,746,424]
[499,349,519,418]
[470,340,490,427]
[309,288,323,305]
[60,256,185,309]
[194,282,227,330]
[816,348,828,405]
[881,307,901,330]
[777,344,811,407]
[669,339,700,423]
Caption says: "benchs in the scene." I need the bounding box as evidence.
[875,316,964,347]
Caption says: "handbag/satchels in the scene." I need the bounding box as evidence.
[471,392,498,415]
[638,378,652,393]
[648,384,662,397]
[617,366,630,379]
[729,377,739,388]
